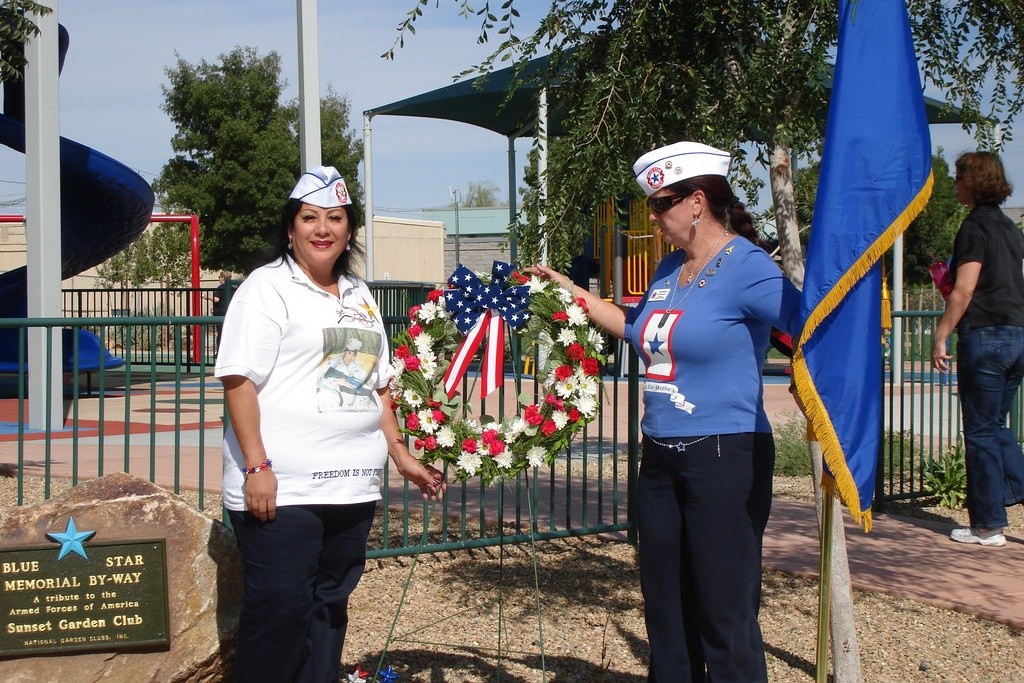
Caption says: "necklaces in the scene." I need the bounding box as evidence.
[658,230,729,330]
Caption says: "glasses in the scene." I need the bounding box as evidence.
[218,275,223,278]
[646,191,692,213]
[955,175,963,182]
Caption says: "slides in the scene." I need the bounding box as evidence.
[0,7,159,377]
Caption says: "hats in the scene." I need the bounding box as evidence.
[288,165,352,208]
[632,141,731,196]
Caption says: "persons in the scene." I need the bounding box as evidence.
[214,165,447,683]
[523,141,889,683]
[205,271,234,358]
[931,150,1024,547]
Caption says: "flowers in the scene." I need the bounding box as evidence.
[387,271,610,494]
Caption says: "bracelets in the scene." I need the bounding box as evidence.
[567,277,573,292]
[242,459,273,474]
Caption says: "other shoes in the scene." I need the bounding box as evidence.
[951,527,1006,546]
[213,353,218,358]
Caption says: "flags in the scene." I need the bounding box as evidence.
[788,0,935,533]
